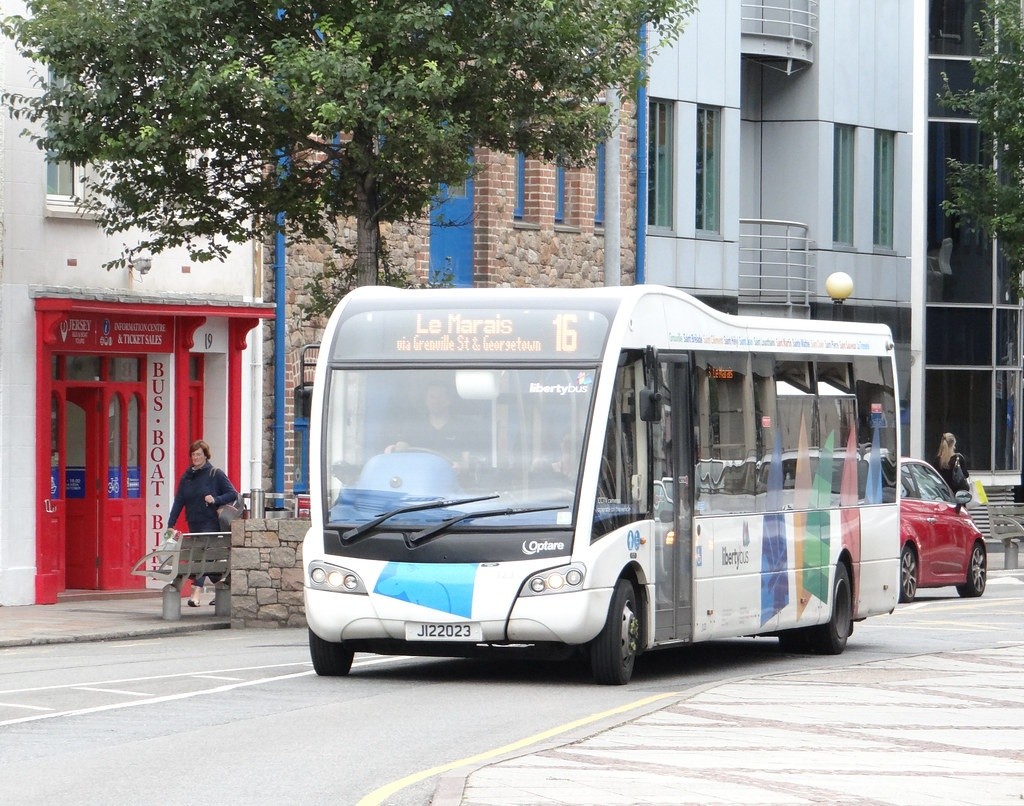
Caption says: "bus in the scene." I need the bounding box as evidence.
[304,286,901,685]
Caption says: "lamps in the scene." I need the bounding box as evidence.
[129,256,152,283]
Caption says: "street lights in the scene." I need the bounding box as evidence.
[826,273,852,319]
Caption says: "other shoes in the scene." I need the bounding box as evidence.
[188,599,200,607]
[209,597,215,605]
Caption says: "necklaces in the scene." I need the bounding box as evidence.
[933,433,970,508]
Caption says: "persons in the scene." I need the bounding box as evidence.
[532,434,577,475]
[168,441,239,607]
[381,381,464,471]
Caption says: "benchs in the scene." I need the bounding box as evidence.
[987,502,1024,570]
[131,532,232,620]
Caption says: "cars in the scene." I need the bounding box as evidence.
[901,458,988,598]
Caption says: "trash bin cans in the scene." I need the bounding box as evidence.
[242,488,295,519]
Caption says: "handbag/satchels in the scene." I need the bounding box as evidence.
[951,454,971,494]
[209,468,246,532]
[157,531,181,566]
